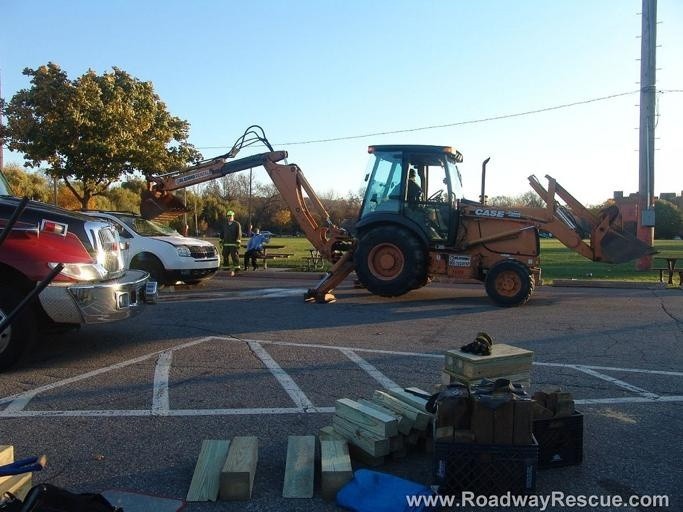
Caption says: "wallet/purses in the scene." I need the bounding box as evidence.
[432,433,539,494]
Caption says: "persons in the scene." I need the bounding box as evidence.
[243,228,265,271]
[388,169,422,201]
[218,210,242,278]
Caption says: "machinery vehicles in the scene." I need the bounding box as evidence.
[139,125,662,305]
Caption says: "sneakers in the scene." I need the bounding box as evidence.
[223,268,241,273]
[244,267,258,271]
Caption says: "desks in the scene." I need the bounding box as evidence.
[305,248,320,271]
[239,244,285,270]
[654,257,683,283]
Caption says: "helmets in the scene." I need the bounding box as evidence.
[226,210,234,216]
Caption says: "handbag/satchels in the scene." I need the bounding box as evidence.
[530,390,575,419]
[434,381,534,445]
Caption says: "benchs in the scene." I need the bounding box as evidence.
[239,253,294,269]
[653,268,682,282]
[302,256,324,271]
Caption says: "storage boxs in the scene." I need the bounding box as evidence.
[437,343,584,499]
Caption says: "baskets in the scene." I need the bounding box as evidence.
[533,410,584,469]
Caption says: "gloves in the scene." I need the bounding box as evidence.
[462,332,493,356]
